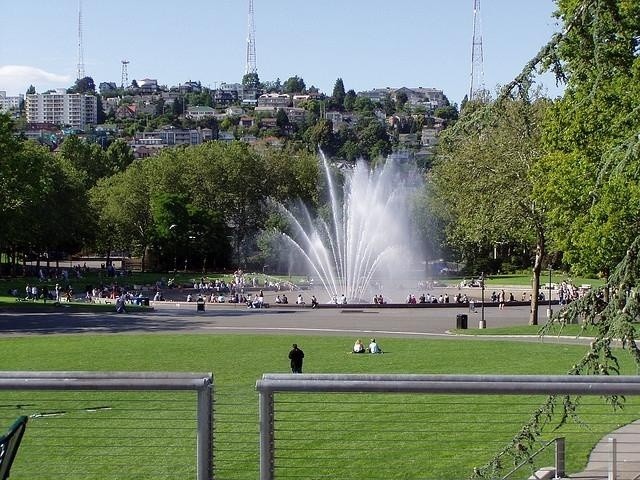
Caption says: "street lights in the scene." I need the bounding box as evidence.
[169,224,180,272]
[546,264,555,319]
[234,223,240,269]
[477,272,487,329]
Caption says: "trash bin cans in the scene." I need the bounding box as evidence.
[131,297,149,306]
[457,314,468,329]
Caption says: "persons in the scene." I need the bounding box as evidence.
[374,295,379,304]
[407,271,587,310]
[378,295,386,304]
[7,261,143,312]
[341,294,347,304]
[298,295,318,309]
[333,294,338,304]
[352,339,365,354]
[367,338,382,354]
[289,343,304,373]
[151,267,288,309]
